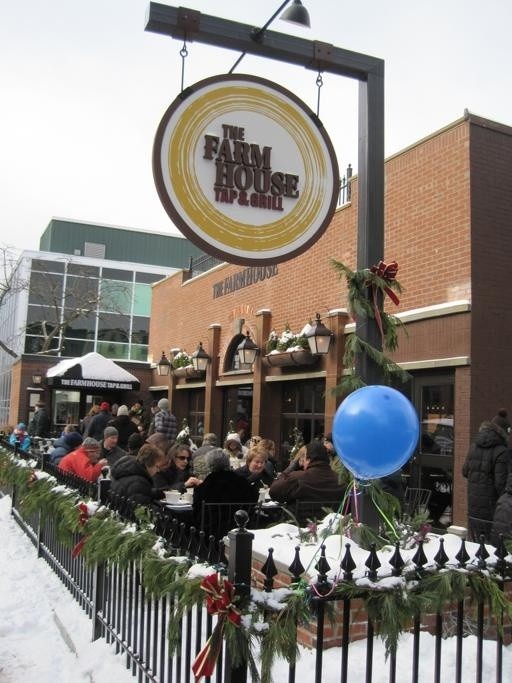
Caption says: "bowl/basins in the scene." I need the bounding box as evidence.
[163,490,181,501]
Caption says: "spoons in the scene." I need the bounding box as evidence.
[260,479,268,489]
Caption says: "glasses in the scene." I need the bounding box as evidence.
[175,455,192,461]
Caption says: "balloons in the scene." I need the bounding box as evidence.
[331,384,423,482]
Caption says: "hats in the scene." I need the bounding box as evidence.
[63,432,83,447]
[227,433,241,443]
[104,426,118,438]
[146,432,167,453]
[100,402,110,410]
[82,437,100,451]
[491,408,508,430]
[158,398,169,408]
[127,433,144,448]
[117,405,128,416]
[18,422,26,429]
[325,433,333,444]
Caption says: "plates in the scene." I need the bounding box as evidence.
[160,499,191,504]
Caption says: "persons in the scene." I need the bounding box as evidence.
[191,433,223,480]
[153,397,177,446]
[82,402,111,440]
[268,442,348,526]
[9,423,31,453]
[152,445,203,489]
[46,440,56,455]
[79,405,99,436]
[192,449,253,538]
[221,432,249,469]
[62,425,76,433]
[97,427,126,466]
[461,408,511,543]
[234,446,273,490]
[255,439,278,479]
[29,403,49,443]
[324,433,350,485]
[58,437,107,483]
[280,445,308,474]
[145,432,168,456]
[111,443,166,517]
[50,433,83,467]
[124,433,170,468]
[493,472,512,554]
[110,404,119,416]
[129,399,150,436]
[106,405,140,450]
[148,401,160,434]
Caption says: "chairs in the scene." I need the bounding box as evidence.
[404,486,432,517]
[200,500,263,541]
[295,500,344,525]
